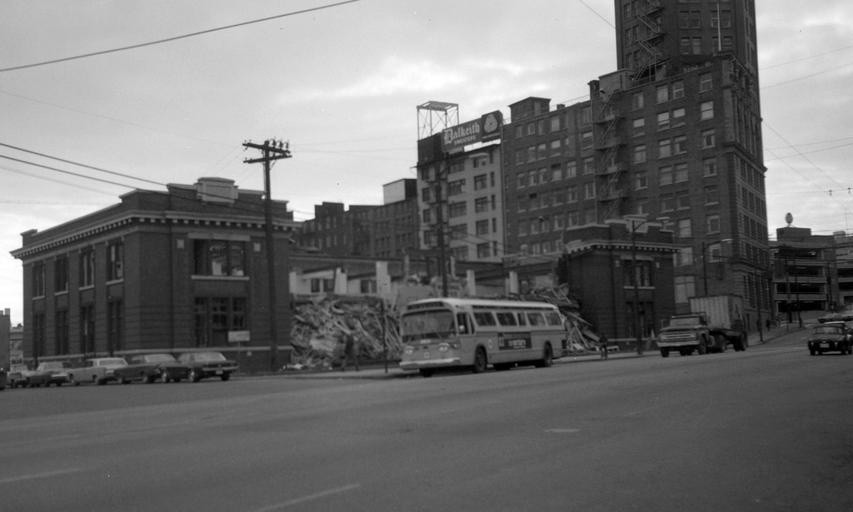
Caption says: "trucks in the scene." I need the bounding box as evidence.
[658,292,747,357]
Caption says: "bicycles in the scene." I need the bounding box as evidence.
[597,343,607,361]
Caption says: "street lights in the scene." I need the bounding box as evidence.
[632,216,668,354]
[754,249,779,341]
[793,250,817,327]
[701,238,735,295]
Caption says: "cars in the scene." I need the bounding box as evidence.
[588,345,619,354]
[0,350,241,390]
[808,310,853,355]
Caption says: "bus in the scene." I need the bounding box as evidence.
[398,299,568,378]
